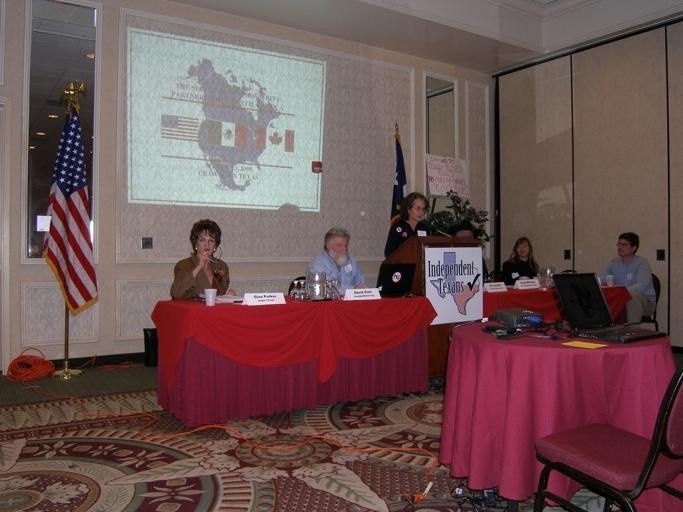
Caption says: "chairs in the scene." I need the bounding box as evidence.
[635,275,662,332]
[524,359,681,512]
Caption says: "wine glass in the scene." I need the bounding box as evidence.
[289,272,338,302]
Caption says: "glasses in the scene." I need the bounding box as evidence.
[411,206,427,212]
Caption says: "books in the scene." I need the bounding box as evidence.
[212,294,243,304]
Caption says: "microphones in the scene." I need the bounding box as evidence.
[421,215,433,230]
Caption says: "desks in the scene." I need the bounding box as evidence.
[152,293,439,429]
[438,322,680,511]
[482,283,629,322]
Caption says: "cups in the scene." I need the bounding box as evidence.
[606,274,615,288]
[533,268,553,289]
[204,289,217,307]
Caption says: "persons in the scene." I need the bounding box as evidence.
[169,218,231,300]
[381,191,430,259]
[305,226,368,297]
[598,231,657,325]
[502,236,539,285]
[454,229,490,282]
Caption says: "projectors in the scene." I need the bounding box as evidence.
[497,306,543,328]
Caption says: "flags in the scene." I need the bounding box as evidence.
[387,126,410,229]
[42,102,97,317]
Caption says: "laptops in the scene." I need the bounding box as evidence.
[551,272,667,344]
[375,263,416,297]
